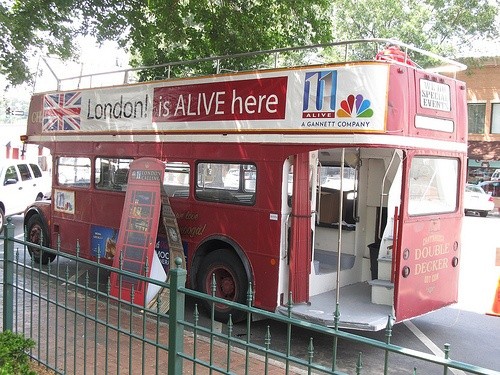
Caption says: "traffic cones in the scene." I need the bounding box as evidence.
[486,279,500,317]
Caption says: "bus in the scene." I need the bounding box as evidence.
[22,39,467,333]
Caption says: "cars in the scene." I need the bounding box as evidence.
[465,184,495,217]
[224,169,251,189]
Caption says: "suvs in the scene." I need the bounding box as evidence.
[0,161,48,234]
